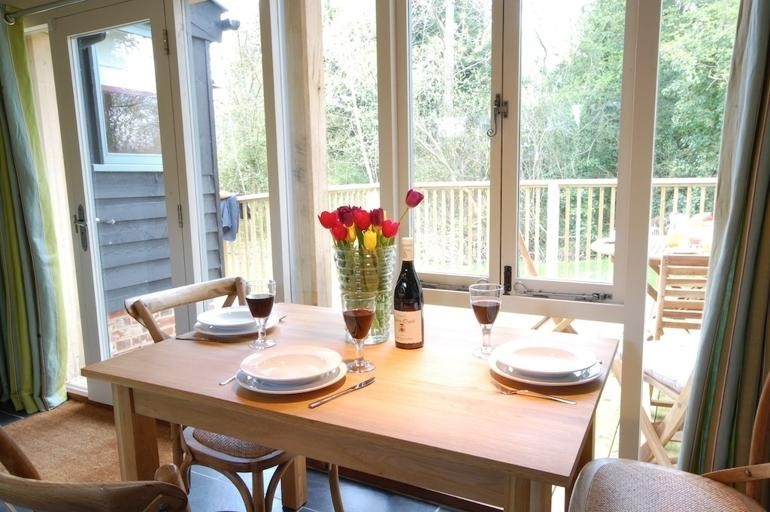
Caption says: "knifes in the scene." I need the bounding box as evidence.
[309,376,377,409]
[175,336,241,347]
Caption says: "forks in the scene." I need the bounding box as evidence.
[493,384,576,407]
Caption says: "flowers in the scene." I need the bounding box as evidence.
[317,187,424,332]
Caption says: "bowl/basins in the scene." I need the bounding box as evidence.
[197,305,270,330]
[495,331,598,375]
[240,345,342,385]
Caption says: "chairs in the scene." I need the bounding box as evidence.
[124,275,345,512]
[656,254,712,338]
[568,456,769,512]
[641,330,701,469]
[0,425,190,512]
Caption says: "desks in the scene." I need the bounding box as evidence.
[79,303,622,511]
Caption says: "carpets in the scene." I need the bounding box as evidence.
[0,398,192,485]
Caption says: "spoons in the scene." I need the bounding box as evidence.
[278,312,288,321]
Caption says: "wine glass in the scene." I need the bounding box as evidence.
[244,277,276,350]
[469,284,504,359]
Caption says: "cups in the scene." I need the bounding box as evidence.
[341,293,376,373]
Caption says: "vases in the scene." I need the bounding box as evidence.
[333,245,394,345]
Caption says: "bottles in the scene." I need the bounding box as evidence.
[393,238,424,350]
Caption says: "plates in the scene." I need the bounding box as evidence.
[235,363,348,395]
[194,318,278,338]
[489,349,604,386]
